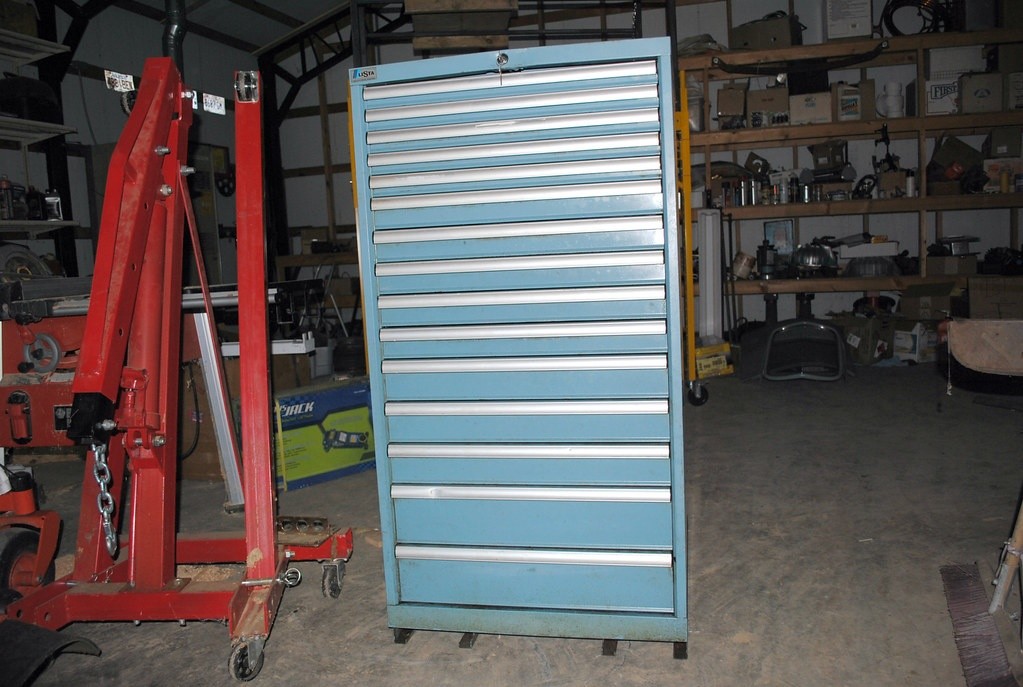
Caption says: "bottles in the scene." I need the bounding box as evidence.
[905,169,916,199]
[756,239,778,275]
[0,174,64,221]
[720,176,823,207]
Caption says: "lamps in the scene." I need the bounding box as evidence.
[699,208,722,338]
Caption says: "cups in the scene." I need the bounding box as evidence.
[732,252,755,278]
[998,169,1010,194]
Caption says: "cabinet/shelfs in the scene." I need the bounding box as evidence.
[677,24,1023,297]
[0,28,81,240]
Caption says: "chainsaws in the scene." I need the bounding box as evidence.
[0,243,54,282]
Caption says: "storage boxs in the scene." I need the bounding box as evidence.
[275,374,375,493]
[746,88,790,129]
[956,71,1003,114]
[987,44,1023,73]
[981,128,1020,159]
[1002,71,1023,112]
[839,241,899,259]
[825,311,896,365]
[787,84,837,126]
[728,14,802,51]
[983,157,1019,177]
[926,69,969,116]
[893,323,938,363]
[837,81,876,123]
[717,82,749,117]
[696,354,727,379]
[897,280,956,321]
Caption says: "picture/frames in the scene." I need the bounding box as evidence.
[764,220,794,256]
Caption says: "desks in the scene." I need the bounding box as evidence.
[281,248,359,338]
